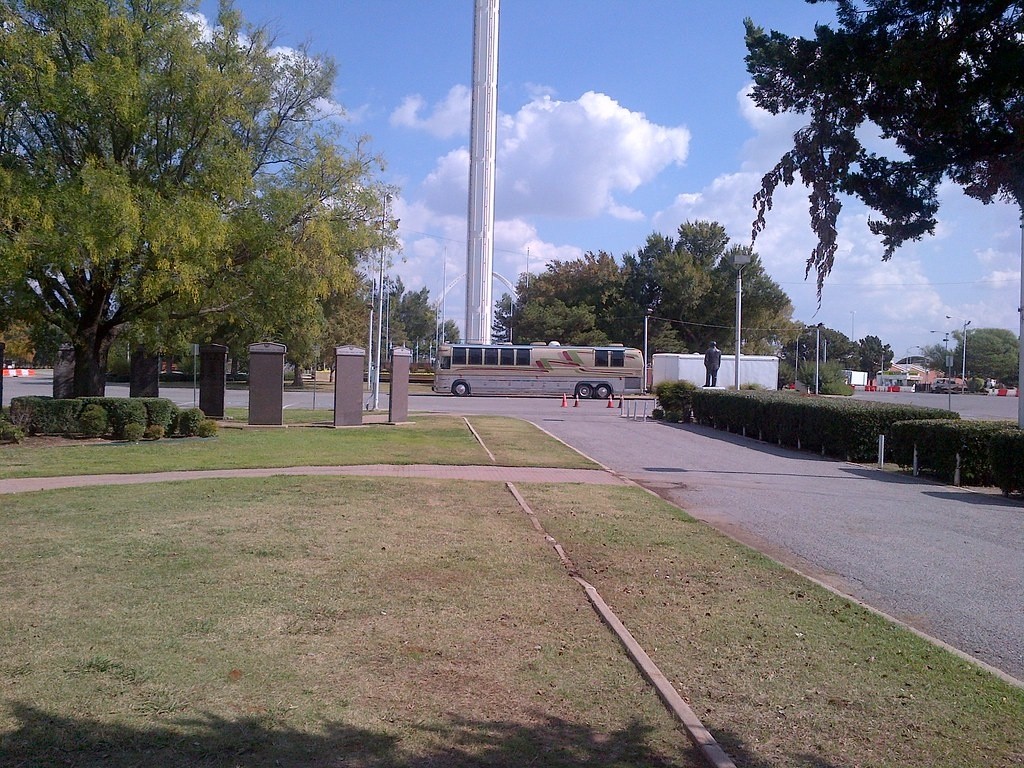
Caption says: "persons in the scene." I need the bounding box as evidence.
[703,342,721,387]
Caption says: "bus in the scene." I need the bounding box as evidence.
[432,340,644,399]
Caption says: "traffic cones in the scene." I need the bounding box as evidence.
[559,392,569,408]
[572,393,581,407]
[617,395,625,409]
[606,394,615,408]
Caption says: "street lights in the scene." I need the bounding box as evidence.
[850,310,856,341]
[931,330,952,411]
[906,345,919,370]
[729,255,752,391]
[373,192,393,409]
[945,315,967,395]
[644,308,654,394]
